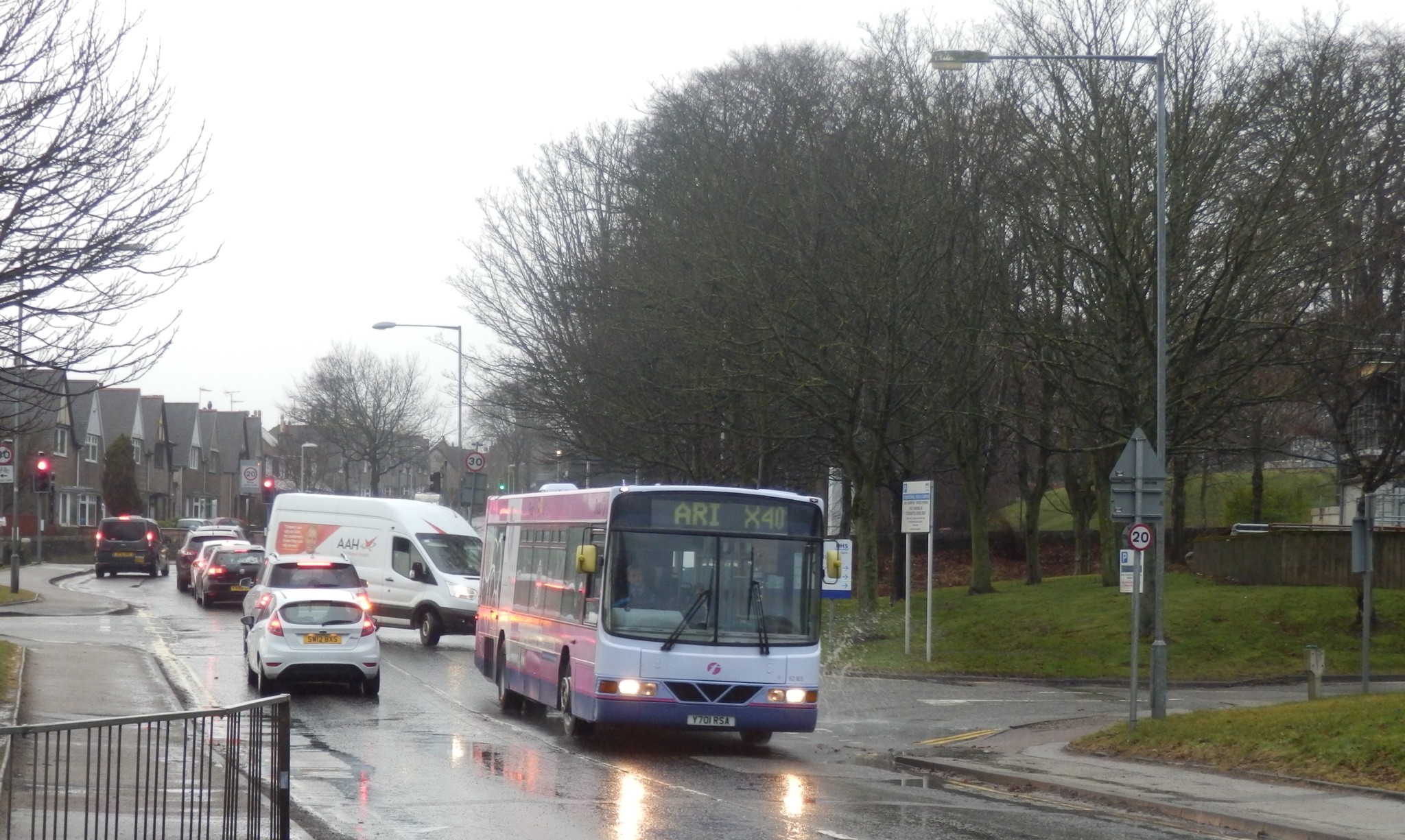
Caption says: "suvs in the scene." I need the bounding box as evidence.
[238,551,369,652]
[176,524,247,592]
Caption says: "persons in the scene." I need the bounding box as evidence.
[625,563,646,600]
[301,526,321,555]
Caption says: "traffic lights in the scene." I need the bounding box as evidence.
[429,471,442,494]
[31,457,52,493]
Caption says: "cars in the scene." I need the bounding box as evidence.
[241,590,381,699]
[190,540,267,608]
[175,516,245,528]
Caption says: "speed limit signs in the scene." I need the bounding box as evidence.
[244,467,258,481]
[1128,524,1152,551]
[465,452,485,471]
[0,445,13,465]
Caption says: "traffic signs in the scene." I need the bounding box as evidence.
[0,465,14,483]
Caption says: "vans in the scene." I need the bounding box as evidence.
[94,515,172,578]
[265,491,485,646]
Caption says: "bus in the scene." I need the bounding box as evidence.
[473,484,842,747]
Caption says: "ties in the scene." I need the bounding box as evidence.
[634,589,640,599]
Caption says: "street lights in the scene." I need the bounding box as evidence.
[10,243,148,595]
[467,442,484,525]
[372,322,462,515]
[300,442,317,492]
[926,49,1167,719]
